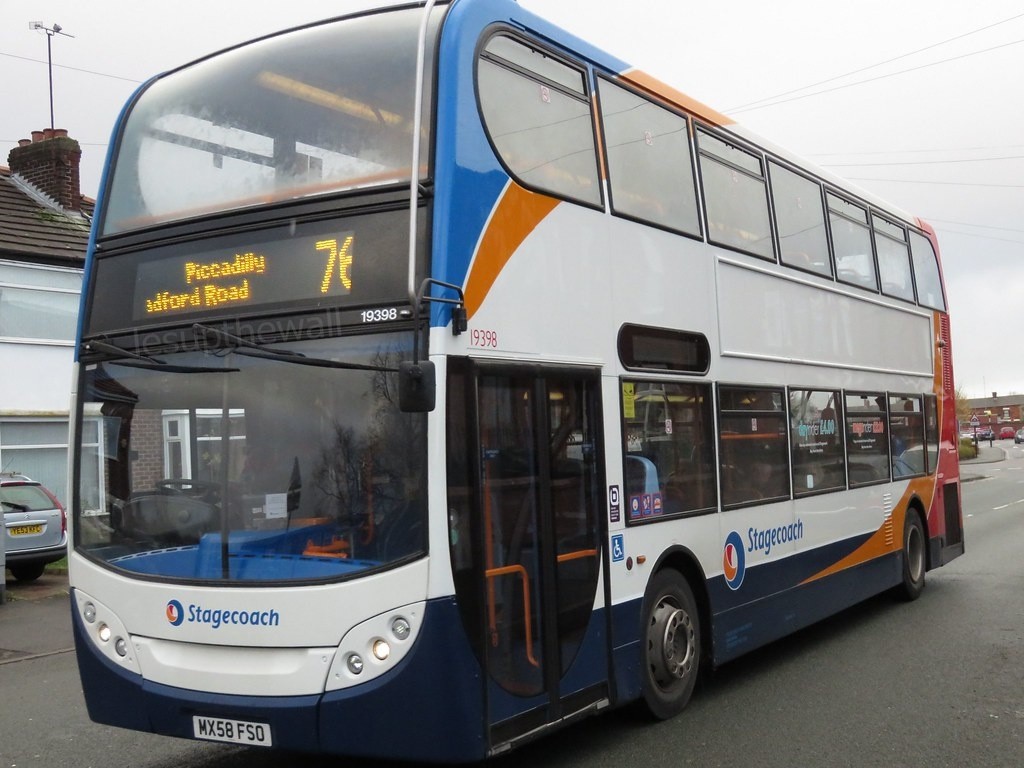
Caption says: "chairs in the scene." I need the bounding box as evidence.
[625,437,882,500]
[523,455,661,579]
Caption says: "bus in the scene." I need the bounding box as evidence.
[64,0,964,768]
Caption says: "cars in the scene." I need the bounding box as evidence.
[999,427,1015,439]
[0,472,68,582]
[962,429,996,442]
[1014,429,1024,444]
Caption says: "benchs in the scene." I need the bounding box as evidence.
[713,226,906,300]
